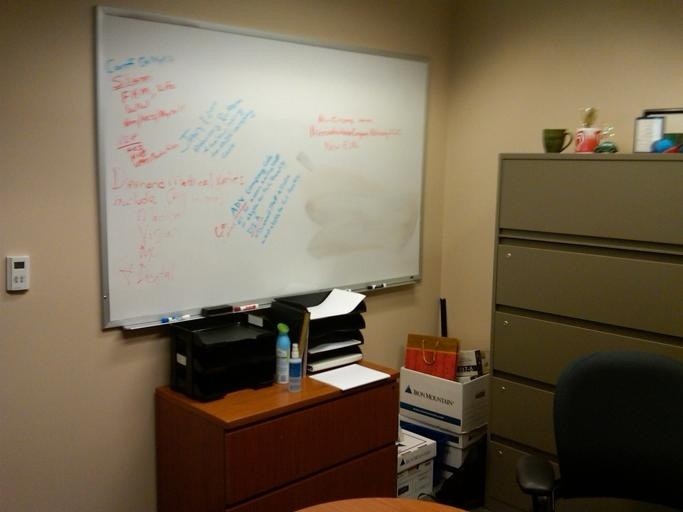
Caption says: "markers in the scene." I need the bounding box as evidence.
[367,282,386,289]
[234,304,259,312]
[161,313,191,323]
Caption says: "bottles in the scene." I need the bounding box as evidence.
[276,322,302,393]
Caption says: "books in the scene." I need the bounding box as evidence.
[456,349,483,377]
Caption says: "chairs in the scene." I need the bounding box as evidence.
[513,350,682,511]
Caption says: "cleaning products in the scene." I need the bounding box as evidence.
[288,343,303,393]
[275,323,291,384]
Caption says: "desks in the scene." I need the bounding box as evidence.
[288,496,471,511]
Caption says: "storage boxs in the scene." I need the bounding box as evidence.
[395,359,492,433]
[393,425,437,499]
[396,412,486,482]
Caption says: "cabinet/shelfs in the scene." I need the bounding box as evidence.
[476,145,683,511]
[146,357,401,510]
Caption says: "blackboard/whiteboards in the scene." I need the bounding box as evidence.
[95,4,431,331]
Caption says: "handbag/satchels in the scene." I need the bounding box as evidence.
[404,332,459,382]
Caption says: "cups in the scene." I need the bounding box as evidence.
[541,127,603,154]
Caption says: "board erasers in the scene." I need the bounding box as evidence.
[199,304,233,316]
